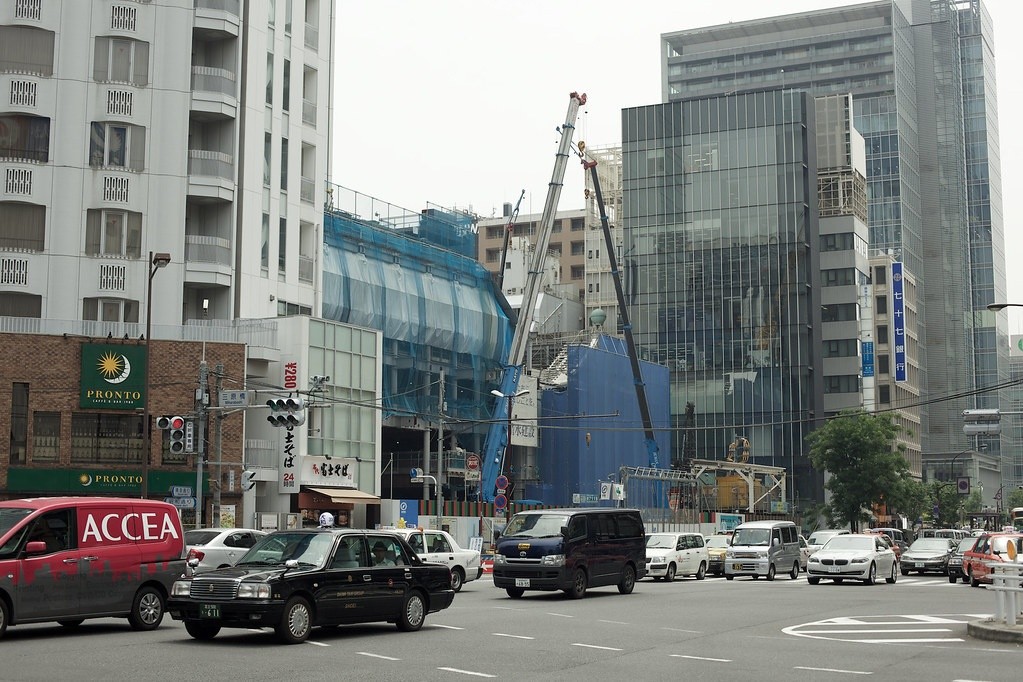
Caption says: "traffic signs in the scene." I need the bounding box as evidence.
[164,486,198,509]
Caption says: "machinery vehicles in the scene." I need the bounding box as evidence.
[581,155,779,523]
[465,89,586,512]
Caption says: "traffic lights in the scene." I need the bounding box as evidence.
[156,416,185,455]
[266,398,305,428]
[241,471,256,491]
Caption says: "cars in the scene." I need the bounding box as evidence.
[645,532,709,581]
[947,526,1023,587]
[169,529,456,641]
[705,535,737,576]
[356,527,483,592]
[901,537,958,575]
[799,530,850,573]
[185,528,287,574]
[806,534,898,584]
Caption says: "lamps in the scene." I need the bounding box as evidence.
[64,332,145,345]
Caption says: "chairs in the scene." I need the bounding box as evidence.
[338,549,360,567]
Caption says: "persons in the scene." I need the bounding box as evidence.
[432,536,444,554]
[370,541,396,567]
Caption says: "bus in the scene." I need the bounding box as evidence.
[1010,507,1023,533]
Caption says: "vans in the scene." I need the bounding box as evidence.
[872,527,909,551]
[493,506,646,598]
[724,519,800,580]
[0,496,189,638]
[935,529,970,545]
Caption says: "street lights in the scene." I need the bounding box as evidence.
[951,444,988,529]
[491,390,530,521]
[142,253,170,500]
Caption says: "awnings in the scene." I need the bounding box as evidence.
[306,487,380,504]
